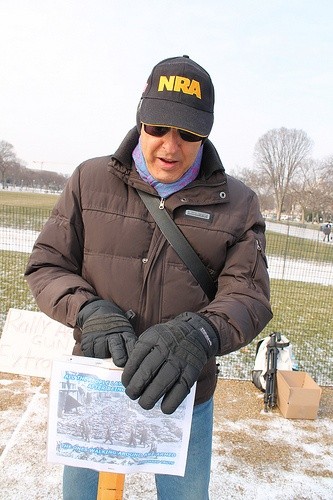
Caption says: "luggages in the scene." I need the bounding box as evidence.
[252,333,298,394]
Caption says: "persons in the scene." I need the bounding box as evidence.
[322,222,331,242]
[25,54,272,498]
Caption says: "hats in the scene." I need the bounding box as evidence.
[136,55,214,147]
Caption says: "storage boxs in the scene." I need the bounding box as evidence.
[277,370,322,420]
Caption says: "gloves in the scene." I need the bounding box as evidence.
[122,311,221,414]
[76,296,138,367]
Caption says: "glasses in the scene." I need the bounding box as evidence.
[144,124,204,142]
